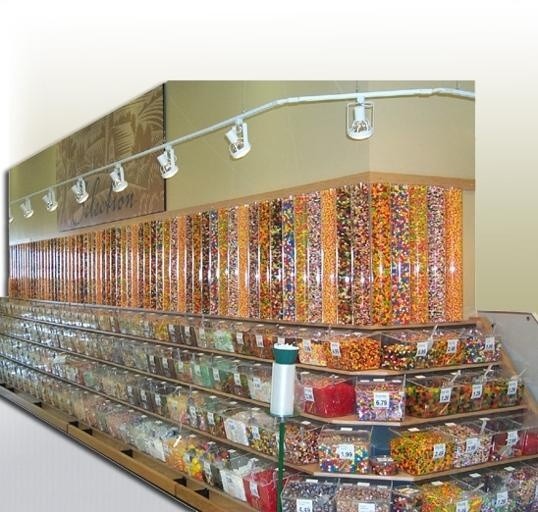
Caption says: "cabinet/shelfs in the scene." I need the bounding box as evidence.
[210,323,538,512]
[0,296,538,512]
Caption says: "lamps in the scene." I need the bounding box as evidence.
[7,94,373,225]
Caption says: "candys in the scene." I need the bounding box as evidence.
[6,180,538,511]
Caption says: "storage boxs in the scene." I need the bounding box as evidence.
[352,375,405,424]
[211,323,382,369]
[406,371,528,416]
[211,355,352,422]
[213,456,280,511]
[317,425,370,472]
[392,466,538,512]
[282,478,392,512]
[382,327,505,365]
[388,414,538,472]
[211,403,317,468]
